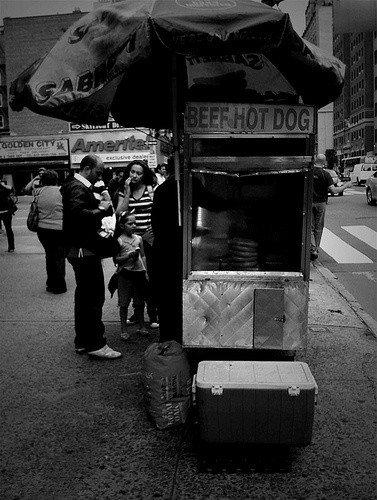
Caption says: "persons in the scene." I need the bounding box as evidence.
[153,163,166,191]
[310,153,352,259]
[24,168,46,198]
[0,183,18,251]
[34,169,70,293]
[60,154,122,359]
[149,156,232,341]
[113,158,159,327]
[112,211,149,341]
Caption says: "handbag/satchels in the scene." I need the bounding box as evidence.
[26,188,43,231]
[107,271,119,298]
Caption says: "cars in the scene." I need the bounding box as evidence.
[366,171,377,206]
[323,168,343,196]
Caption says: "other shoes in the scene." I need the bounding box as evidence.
[75,347,86,354]
[88,345,123,359]
[137,327,150,335]
[150,318,159,328]
[125,314,144,324]
[120,331,129,340]
[311,252,318,259]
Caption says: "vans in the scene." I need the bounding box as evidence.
[349,163,377,186]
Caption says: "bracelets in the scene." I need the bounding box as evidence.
[125,195,129,198]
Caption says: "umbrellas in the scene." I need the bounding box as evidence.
[10,0,348,176]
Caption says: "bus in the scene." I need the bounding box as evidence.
[339,155,377,180]
